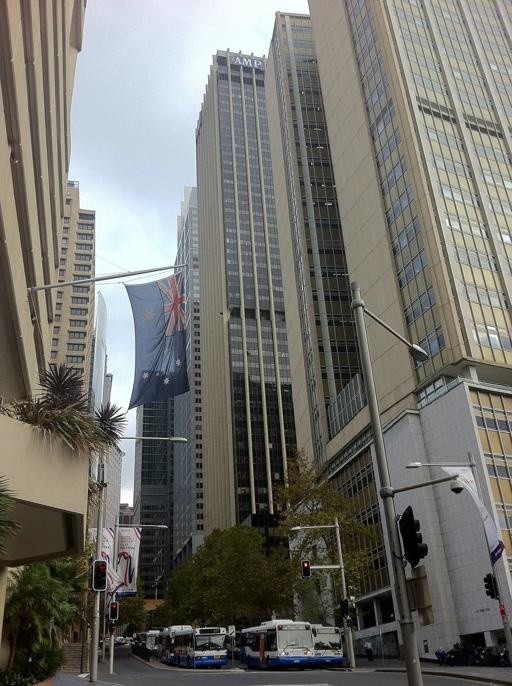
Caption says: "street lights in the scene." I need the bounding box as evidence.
[91,375,187,681]
[405,451,511,664]
[109,514,168,676]
[351,282,430,685]
[289,517,356,668]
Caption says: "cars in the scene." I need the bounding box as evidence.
[100,636,133,651]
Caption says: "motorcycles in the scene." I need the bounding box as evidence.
[435,642,511,667]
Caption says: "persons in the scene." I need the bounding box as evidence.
[364,637,374,662]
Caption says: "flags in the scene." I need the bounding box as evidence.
[124,267,190,409]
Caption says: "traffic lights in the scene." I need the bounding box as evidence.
[399,505,428,569]
[301,560,311,579]
[109,600,120,621]
[482,572,499,599]
[92,560,107,591]
[341,599,349,616]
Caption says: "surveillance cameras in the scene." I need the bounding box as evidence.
[450,480,464,494]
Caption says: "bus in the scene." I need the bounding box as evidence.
[131,619,345,669]
